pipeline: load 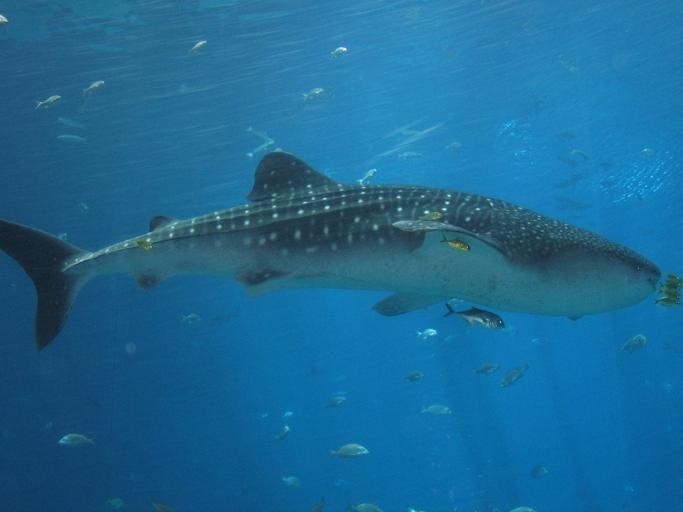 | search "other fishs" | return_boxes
[190,40,207,53]
[103,495,123,508]
[301,87,325,102]
[444,301,505,330]
[444,142,463,150]
[440,232,471,253]
[417,211,445,221]
[358,169,377,186]
[621,334,649,353]
[654,271,683,307]
[180,313,200,323]
[512,507,534,511]
[244,125,275,158]
[33,79,105,146]
[257,394,383,511]
[529,464,548,478]
[403,362,527,416]
[330,47,347,58]
[148,497,173,512]
[56,432,95,448]
[416,328,437,338]
